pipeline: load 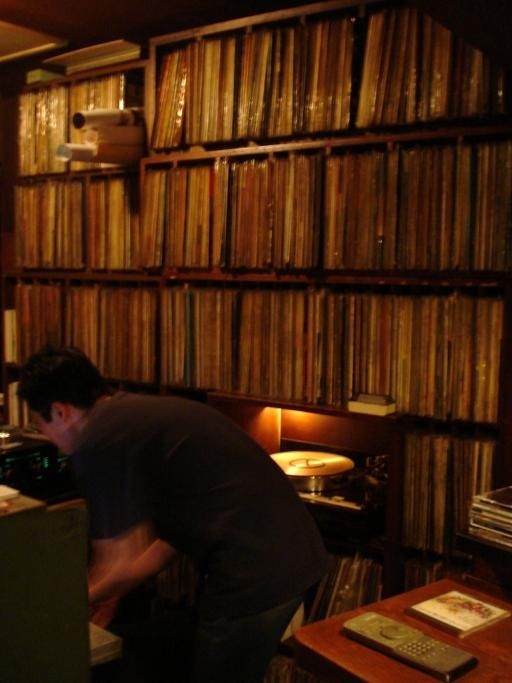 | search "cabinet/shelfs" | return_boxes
[0,0,512,682]
